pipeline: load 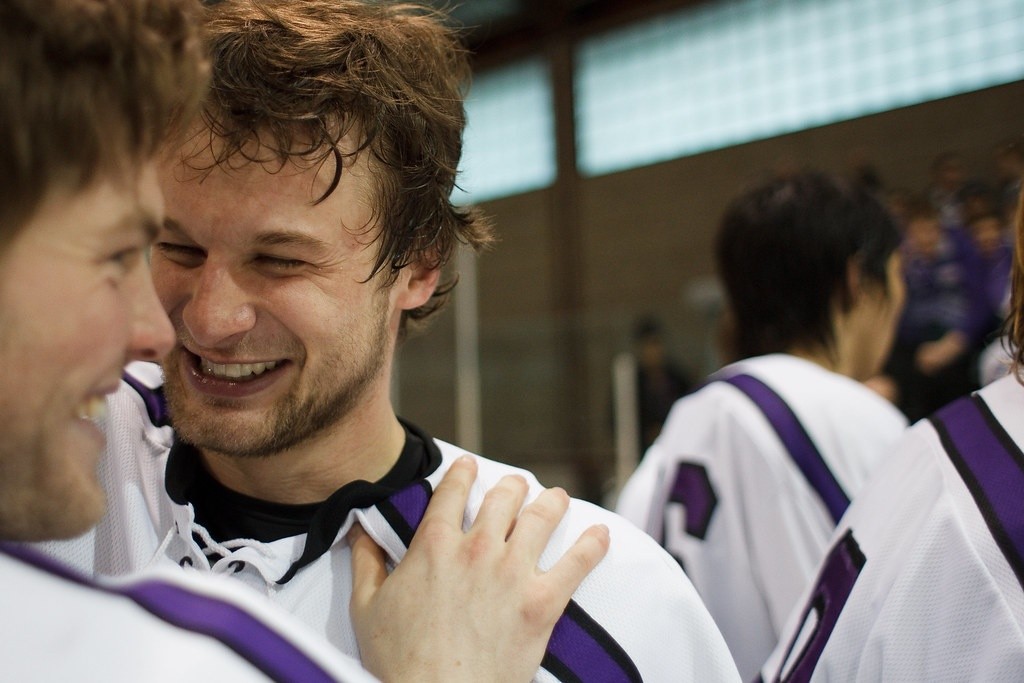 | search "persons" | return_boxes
[752,186,1024,683]
[859,144,1024,425]
[606,318,696,463]
[36,4,743,683]
[617,180,911,683]
[0,0,610,682]
